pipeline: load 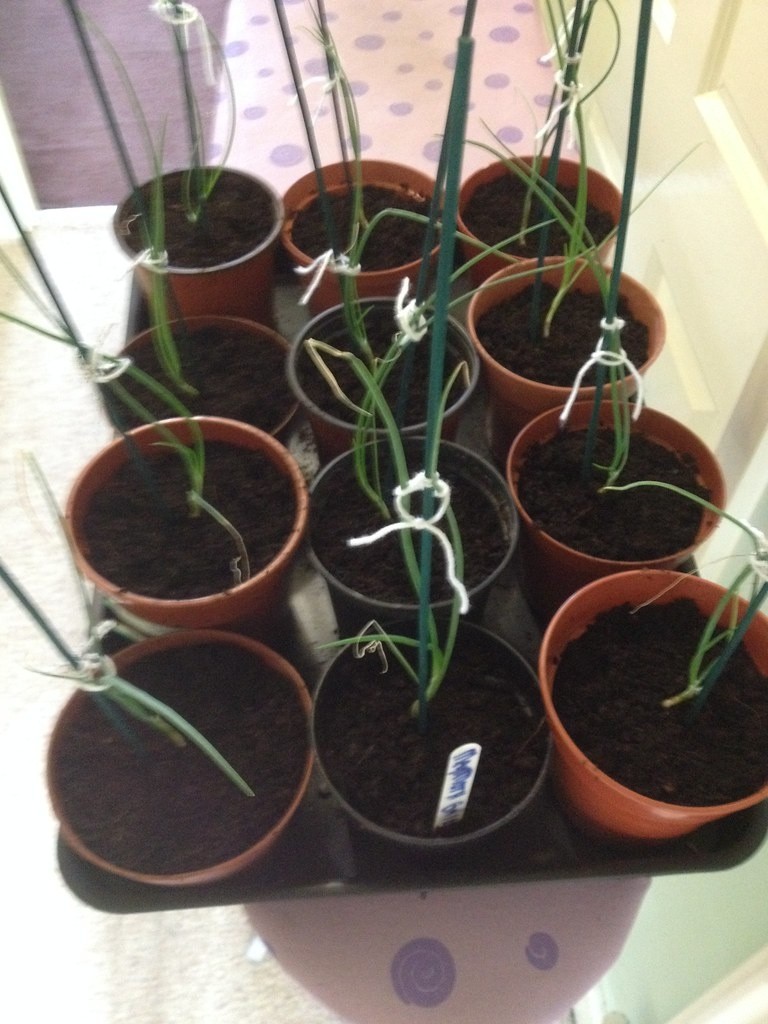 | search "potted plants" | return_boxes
[0,0,768,886]
